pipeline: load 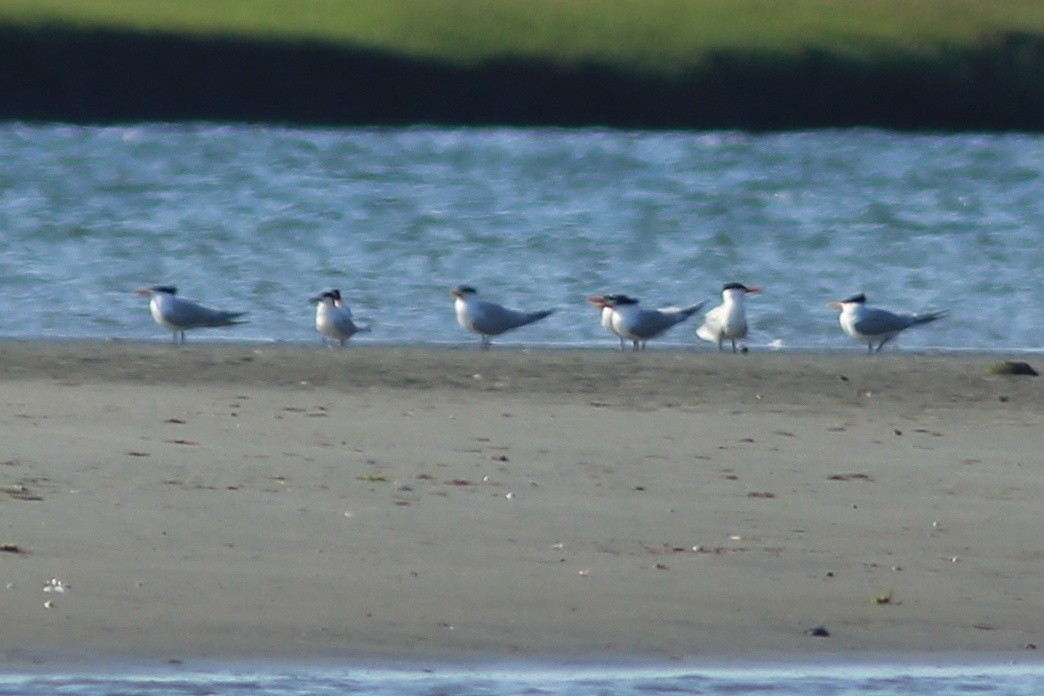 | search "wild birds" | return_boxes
[308,288,371,348]
[133,284,251,345]
[696,283,762,353]
[587,292,713,354]
[452,285,557,347]
[826,292,950,353]
